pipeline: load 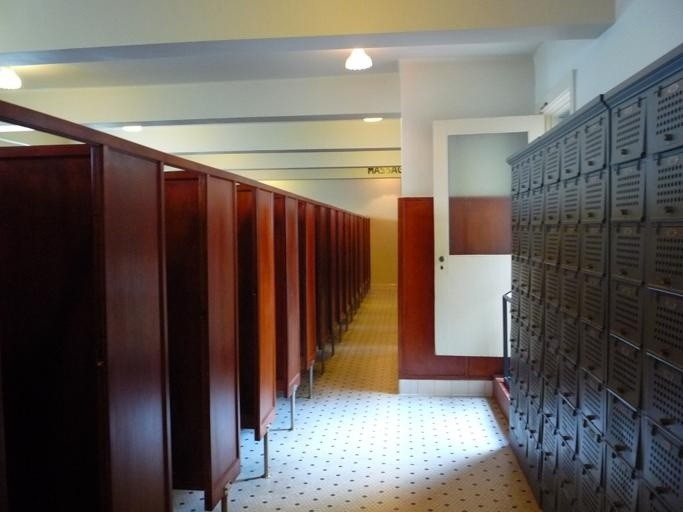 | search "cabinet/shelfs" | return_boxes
[506,44,683,511]
